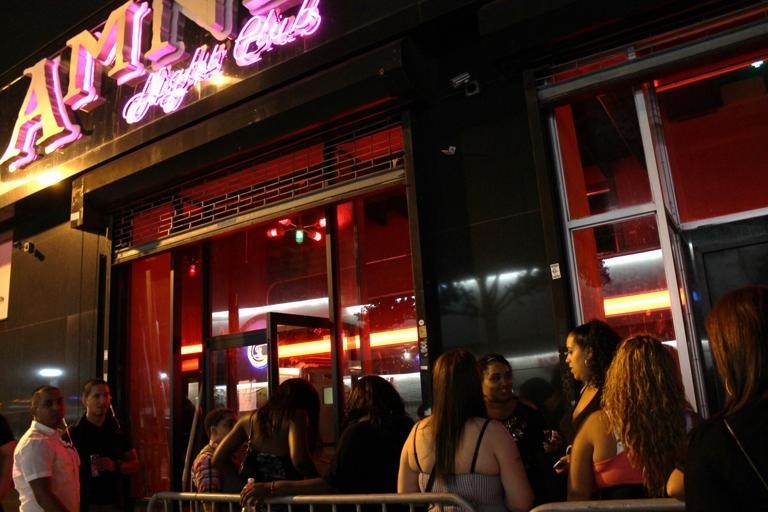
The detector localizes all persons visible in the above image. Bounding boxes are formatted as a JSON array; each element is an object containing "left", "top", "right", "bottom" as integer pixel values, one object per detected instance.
[
  {"left": 63, "top": 378, "right": 140, "bottom": 511},
  {"left": 12, "top": 385, "right": 81, "bottom": 511},
  {"left": 0, "top": 411, "right": 19, "bottom": 512}
]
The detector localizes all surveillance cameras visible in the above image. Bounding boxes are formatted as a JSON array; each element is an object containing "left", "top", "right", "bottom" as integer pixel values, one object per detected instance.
[
  {"left": 450, "top": 72, "right": 472, "bottom": 89},
  {"left": 13, "top": 241, "right": 23, "bottom": 248}
]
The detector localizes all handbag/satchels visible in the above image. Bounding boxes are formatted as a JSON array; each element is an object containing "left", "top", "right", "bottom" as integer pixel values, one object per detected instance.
[{"left": 239, "top": 440, "right": 295, "bottom": 482}]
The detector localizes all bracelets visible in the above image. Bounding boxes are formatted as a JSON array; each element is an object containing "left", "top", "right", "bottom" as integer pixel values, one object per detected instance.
[{"left": 114, "top": 462, "right": 121, "bottom": 475}]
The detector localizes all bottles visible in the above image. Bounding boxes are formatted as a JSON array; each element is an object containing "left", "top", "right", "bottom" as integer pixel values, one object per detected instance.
[{"left": 240, "top": 477, "right": 256, "bottom": 512}]
